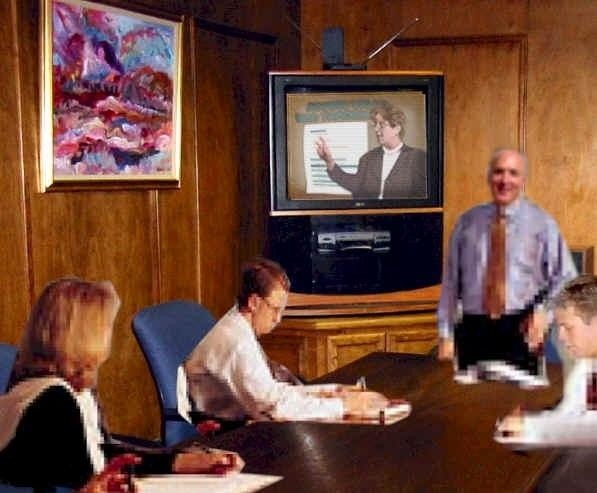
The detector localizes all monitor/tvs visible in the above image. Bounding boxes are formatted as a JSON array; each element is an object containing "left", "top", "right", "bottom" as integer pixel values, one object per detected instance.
[{"left": 269, "top": 70, "right": 444, "bottom": 216}]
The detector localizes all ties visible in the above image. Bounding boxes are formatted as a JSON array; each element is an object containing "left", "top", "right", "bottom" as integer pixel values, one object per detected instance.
[{"left": 481, "top": 205, "right": 507, "bottom": 320}]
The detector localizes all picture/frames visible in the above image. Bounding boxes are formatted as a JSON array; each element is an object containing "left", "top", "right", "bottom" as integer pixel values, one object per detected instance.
[{"left": 36, "top": 0, "right": 185, "bottom": 192}]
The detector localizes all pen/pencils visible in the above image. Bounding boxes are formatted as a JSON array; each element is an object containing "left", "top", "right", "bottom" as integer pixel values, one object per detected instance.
[
  {"left": 193, "top": 441, "right": 211, "bottom": 454},
  {"left": 128, "top": 464, "right": 135, "bottom": 493}
]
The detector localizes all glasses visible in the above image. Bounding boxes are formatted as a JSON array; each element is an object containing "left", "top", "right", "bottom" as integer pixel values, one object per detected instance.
[{"left": 371, "top": 120, "right": 392, "bottom": 129}]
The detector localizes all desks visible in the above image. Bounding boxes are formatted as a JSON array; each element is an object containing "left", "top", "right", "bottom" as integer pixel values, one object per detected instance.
[{"left": 115, "top": 349, "right": 596, "bottom": 491}]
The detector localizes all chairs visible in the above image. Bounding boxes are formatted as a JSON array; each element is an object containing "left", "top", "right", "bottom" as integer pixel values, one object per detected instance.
[
  {"left": 0, "top": 339, "right": 157, "bottom": 493},
  {"left": 132, "top": 297, "right": 272, "bottom": 447}
]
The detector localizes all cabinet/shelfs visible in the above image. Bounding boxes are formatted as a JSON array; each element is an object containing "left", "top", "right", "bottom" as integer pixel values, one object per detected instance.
[{"left": 253, "top": 282, "right": 480, "bottom": 394}]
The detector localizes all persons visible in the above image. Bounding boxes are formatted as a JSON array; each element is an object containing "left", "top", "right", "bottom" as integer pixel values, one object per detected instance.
[
  {"left": 437, "top": 149, "right": 579, "bottom": 373},
  {"left": 495, "top": 273, "right": 597, "bottom": 445},
  {"left": 314, "top": 105, "right": 426, "bottom": 198},
  {"left": 185, "top": 259, "right": 390, "bottom": 422},
  {"left": 0, "top": 454, "right": 141, "bottom": 493},
  {"left": 0, "top": 277, "right": 245, "bottom": 485}
]
